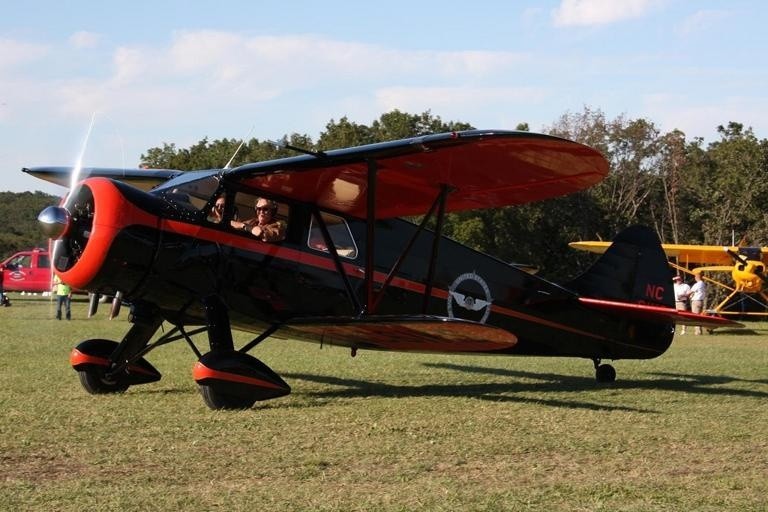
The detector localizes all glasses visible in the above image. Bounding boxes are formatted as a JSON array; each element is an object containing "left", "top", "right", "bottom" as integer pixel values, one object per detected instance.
[{"left": 255, "top": 206, "right": 268, "bottom": 211}]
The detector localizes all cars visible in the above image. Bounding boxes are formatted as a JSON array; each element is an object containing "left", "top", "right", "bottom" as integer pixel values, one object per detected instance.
[{"left": 0, "top": 247, "right": 53, "bottom": 292}]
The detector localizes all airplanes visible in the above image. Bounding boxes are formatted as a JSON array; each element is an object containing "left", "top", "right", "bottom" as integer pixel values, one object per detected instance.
[
  {"left": 568, "top": 233, "right": 768, "bottom": 318},
  {"left": 21, "top": 130, "right": 747, "bottom": 410}
]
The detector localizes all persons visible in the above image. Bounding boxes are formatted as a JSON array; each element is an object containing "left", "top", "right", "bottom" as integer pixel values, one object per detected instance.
[
  {"left": 53, "top": 274, "right": 72, "bottom": 319},
  {"left": 207, "top": 196, "right": 284, "bottom": 242},
  {"left": 673, "top": 272, "right": 707, "bottom": 335}
]
